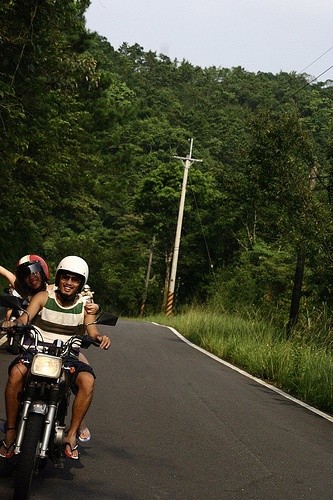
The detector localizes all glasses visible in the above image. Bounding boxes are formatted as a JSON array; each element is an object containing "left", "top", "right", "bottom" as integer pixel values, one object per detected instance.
[{"left": 59, "top": 273, "right": 82, "bottom": 282}]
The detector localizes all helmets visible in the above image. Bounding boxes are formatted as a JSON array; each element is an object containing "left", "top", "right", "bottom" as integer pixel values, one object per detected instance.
[
  {"left": 54, "top": 256, "right": 89, "bottom": 294},
  {"left": 84, "top": 284, "right": 89, "bottom": 292},
  {"left": 16, "top": 255, "right": 50, "bottom": 292}
]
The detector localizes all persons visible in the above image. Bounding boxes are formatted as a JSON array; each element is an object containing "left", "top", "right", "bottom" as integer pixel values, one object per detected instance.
[{"left": 0, "top": 254, "right": 111, "bottom": 459}]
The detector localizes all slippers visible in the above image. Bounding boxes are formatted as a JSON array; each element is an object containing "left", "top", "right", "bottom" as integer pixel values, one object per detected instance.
[
  {"left": 76, "top": 422, "right": 91, "bottom": 442},
  {"left": 0, "top": 439, "right": 17, "bottom": 458},
  {"left": 61, "top": 436, "right": 79, "bottom": 460}
]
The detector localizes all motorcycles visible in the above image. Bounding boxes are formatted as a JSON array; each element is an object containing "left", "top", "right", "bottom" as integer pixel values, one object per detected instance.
[{"left": 0, "top": 294, "right": 118, "bottom": 500}]
